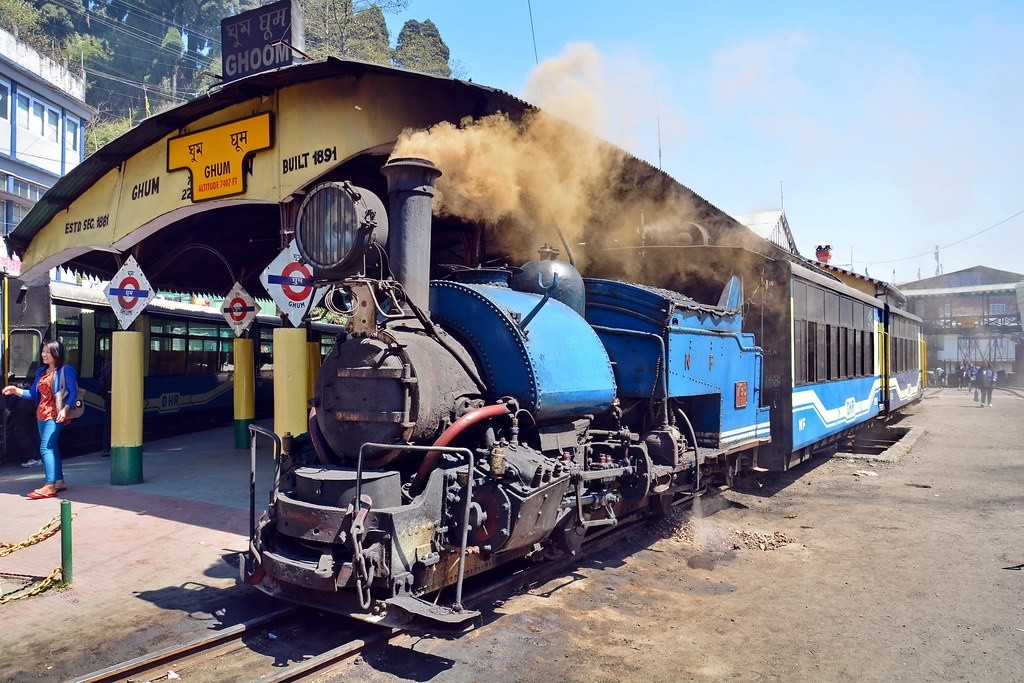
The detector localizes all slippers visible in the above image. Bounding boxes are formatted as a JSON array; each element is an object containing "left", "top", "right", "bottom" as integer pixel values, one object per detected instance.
[
  {"left": 28, "top": 489, "right": 57, "bottom": 498},
  {"left": 56, "top": 488, "right": 67, "bottom": 493}
]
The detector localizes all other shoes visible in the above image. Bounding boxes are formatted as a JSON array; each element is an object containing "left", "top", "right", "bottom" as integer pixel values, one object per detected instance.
[
  {"left": 981, "top": 404, "right": 986, "bottom": 407},
  {"left": 988, "top": 403, "right": 993, "bottom": 407}
]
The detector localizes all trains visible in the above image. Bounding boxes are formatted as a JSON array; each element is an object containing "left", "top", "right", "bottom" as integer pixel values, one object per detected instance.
[
  {"left": 222, "top": 155, "right": 928, "bottom": 634},
  {"left": 0, "top": 275, "right": 346, "bottom": 463}
]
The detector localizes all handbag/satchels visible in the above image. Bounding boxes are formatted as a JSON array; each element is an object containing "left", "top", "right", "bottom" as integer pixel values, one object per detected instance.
[
  {"left": 974, "top": 392, "right": 980, "bottom": 402},
  {"left": 56, "top": 364, "right": 86, "bottom": 419}
]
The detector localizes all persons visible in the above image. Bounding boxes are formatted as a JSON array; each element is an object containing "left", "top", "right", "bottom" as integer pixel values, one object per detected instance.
[
  {"left": 954, "top": 360, "right": 997, "bottom": 407},
  {"left": 0, "top": 338, "right": 79, "bottom": 500},
  {"left": 926, "top": 365, "right": 944, "bottom": 387}
]
